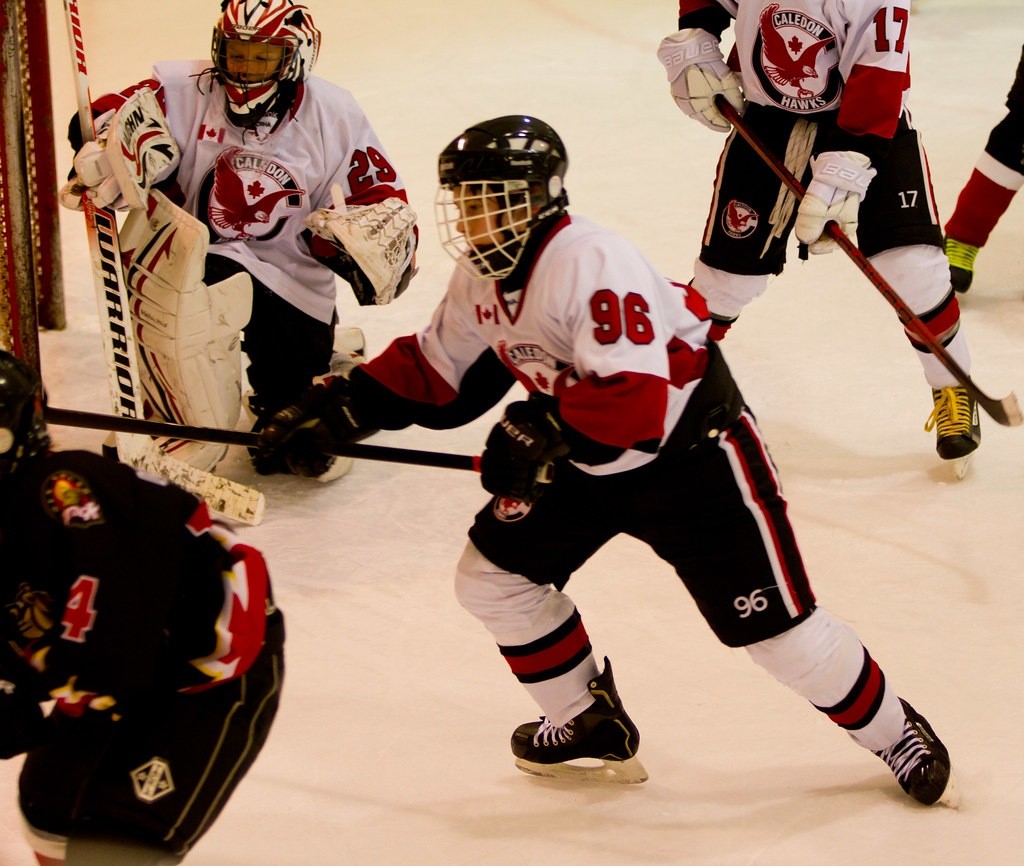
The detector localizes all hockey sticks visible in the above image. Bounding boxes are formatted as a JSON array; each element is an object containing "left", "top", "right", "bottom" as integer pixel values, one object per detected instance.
[
  {"left": 60, "top": 1, "right": 266, "bottom": 525},
  {"left": 42, "top": 405, "right": 554, "bottom": 482},
  {"left": 715, "top": 94, "right": 1024, "bottom": 428}
]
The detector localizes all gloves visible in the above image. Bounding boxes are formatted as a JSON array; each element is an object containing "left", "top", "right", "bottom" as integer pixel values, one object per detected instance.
[
  {"left": 248, "top": 374, "right": 379, "bottom": 474},
  {"left": 794, "top": 151, "right": 876, "bottom": 254},
  {"left": 658, "top": 28, "right": 745, "bottom": 132},
  {"left": 478, "top": 392, "right": 575, "bottom": 502}
]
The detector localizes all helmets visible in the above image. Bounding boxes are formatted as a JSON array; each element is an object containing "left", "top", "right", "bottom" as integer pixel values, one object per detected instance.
[
  {"left": 215, "top": 0, "right": 322, "bottom": 130},
  {"left": 438, "top": 115, "right": 568, "bottom": 207}
]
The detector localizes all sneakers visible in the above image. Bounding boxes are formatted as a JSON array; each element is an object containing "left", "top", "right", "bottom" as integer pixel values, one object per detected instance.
[
  {"left": 510, "top": 656, "right": 649, "bottom": 784},
  {"left": 925, "top": 375, "right": 981, "bottom": 480},
  {"left": 945, "top": 234, "right": 978, "bottom": 305},
  {"left": 871, "top": 697, "right": 962, "bottom": 810}
]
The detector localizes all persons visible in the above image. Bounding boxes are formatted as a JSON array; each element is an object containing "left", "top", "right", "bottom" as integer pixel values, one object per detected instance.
[
  {"left": 945, "top": 43, "right": 1024, "bottom": 293},
  {"left": 248, "top": 115, "right": 951, "bottom": 807},
  {"left": 66, "top": 0, "right": 419, "bottom": 483},
  {"left": 0, "top": 350, "right": 285, "bottom": 866},
  {"left": 657, "top": 0, "right": 982, "bottom": 481}
]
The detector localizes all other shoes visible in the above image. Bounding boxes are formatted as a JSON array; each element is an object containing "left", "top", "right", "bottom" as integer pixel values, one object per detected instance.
[{"left": 333, "top": 325, "right": 367, "bottom": 371}]
